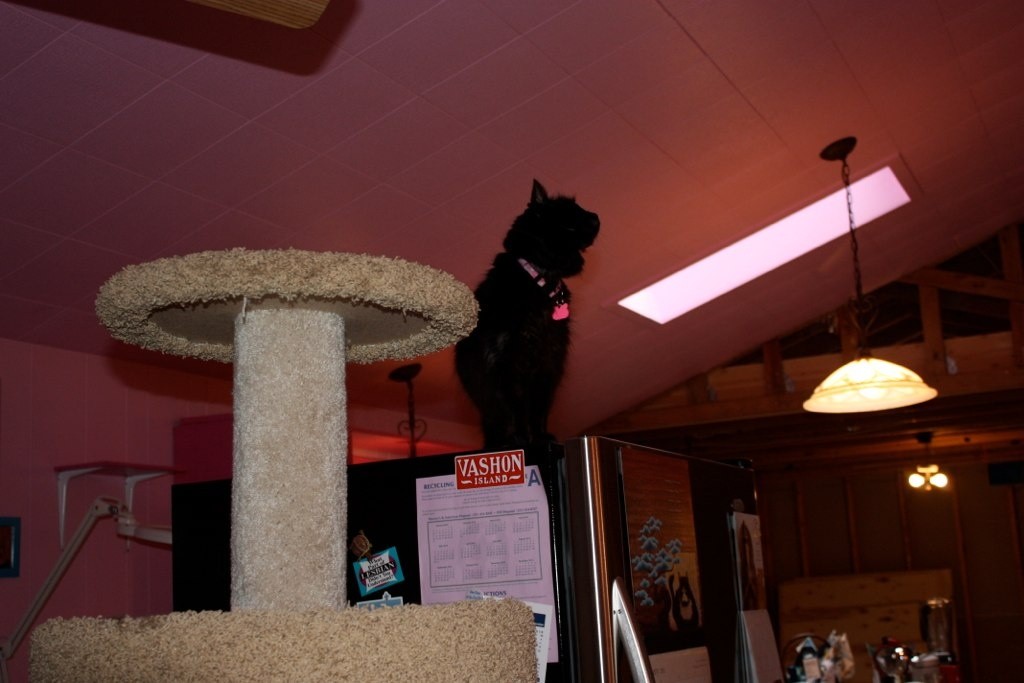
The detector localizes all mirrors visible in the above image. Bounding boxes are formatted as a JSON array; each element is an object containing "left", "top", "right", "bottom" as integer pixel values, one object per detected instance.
[{"left": 0, "top": 517, "right": 20, "bottom": 577}]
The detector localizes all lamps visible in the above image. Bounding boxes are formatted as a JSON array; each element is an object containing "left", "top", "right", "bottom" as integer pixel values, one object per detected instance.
[
  {"left": 908, "top": 430, "right": 948, "bottom": 491},
  {"left": 801, "top": 134, "right": 938, "bottom": 413}
]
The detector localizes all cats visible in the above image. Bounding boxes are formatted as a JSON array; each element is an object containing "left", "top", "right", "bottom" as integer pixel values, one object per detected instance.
[{"left": 455, "top": 178, "right": 602, "bottom": 464}]
